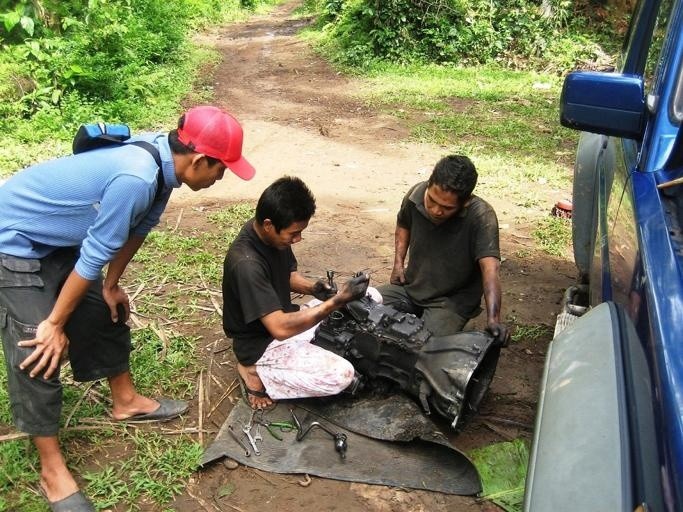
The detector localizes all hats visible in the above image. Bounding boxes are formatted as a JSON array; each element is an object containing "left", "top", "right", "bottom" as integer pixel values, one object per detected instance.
[{"left": 176, "top": 104, "right": 256, "bottom": 182}]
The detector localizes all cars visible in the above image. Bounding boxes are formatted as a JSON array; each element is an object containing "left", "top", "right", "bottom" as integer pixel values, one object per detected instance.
[{"left": 520, "top": 1, "right": 682, "bottom": 511}]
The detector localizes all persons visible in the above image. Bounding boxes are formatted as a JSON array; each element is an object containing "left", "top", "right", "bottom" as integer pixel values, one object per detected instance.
[
  {"left": 221, "top": 175, "right": 382, "bottom": 411},
  {"left": 372, "top": 154, "right": 512, "bottom": 350},
  {"left": 0, "top": 104, "right": 256, "bottom": 510}
]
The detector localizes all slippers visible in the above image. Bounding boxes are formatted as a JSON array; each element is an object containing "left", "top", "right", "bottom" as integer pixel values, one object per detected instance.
[
  {"left": 128, "top": 397, "right": 191, "bottom": 422},
  {"left": 37, "top": 484, "right": 96, "bottom": 512},
  {"left": 238, "top": 372, "right": 274, "bottom": 412}
]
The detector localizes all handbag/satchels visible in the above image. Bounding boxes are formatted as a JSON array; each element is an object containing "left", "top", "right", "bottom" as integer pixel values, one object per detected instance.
[{"left": 71, "top": 121, "right": 132, "bottom": 155}]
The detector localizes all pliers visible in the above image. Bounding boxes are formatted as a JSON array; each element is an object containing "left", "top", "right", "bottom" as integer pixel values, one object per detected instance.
[{"left": 255, "top": 417, "right": 297, "bottom": 441}]
